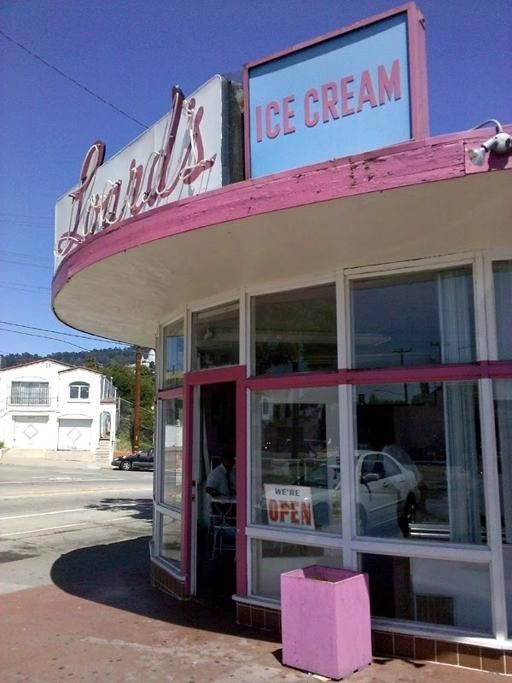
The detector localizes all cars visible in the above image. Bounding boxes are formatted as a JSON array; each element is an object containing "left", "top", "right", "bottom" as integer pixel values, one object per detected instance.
[
  {"left": 290, "top": 450, "right": 420, "bottom": 538},
  {"left": 112, "top": 448, "right": 155, "bottom": 471}
]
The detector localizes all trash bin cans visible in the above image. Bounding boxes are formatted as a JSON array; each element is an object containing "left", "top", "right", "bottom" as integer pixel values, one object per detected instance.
[{"left": 280, "top": 564, "right": 373, "bottom": 681}]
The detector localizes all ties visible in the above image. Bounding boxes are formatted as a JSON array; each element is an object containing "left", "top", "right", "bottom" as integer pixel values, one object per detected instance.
[{"left": 226, "top": 472, "right": 234, "bottom": 490}]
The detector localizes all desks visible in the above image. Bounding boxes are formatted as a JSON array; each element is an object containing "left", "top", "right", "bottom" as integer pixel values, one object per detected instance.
[{"left": 212, "top": 493, "right": 237, "bottom": 554}]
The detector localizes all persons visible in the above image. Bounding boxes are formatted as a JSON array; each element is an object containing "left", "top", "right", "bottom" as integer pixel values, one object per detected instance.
[{"left": 205, "top": 451, "right": 236, "bottom": 526}]
